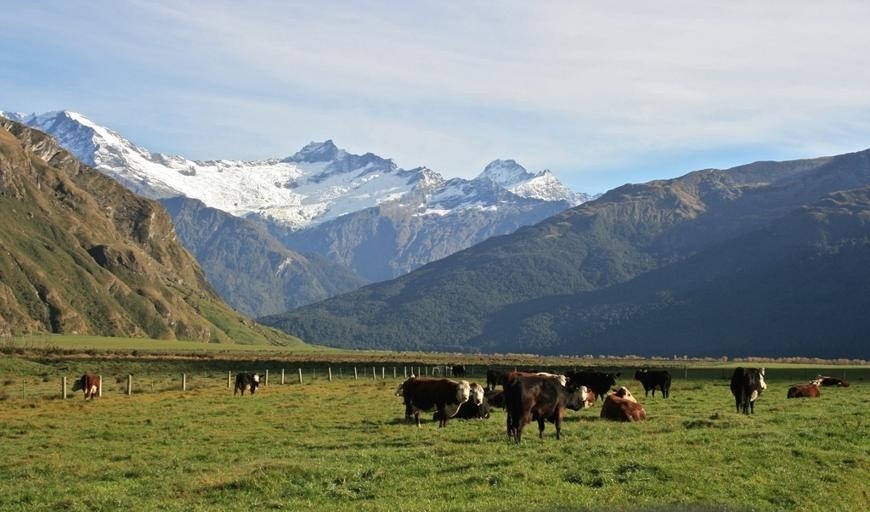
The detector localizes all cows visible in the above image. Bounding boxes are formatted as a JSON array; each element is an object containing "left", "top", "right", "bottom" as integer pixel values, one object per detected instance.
[
  {"left": 401, "top": 368, "right": 623, "bottom": 443},
  {"left": 634, "top": 367, "right": 672, "bottom": 399},
  {"left": 729, "top": 367, "right": 767, "bottom": 414},
  {"left": 787, "top": 378, "right": 821, "bottom": 397},
  {"left": 71, "top": 373, "right": 102, "bottom": 401},
  {"left": 232, "top": 371, "right": 264, "bottom": 396},
  {"left": 602, "top": 387, "right": 648, "bottom": 422},
  {"left": 817, "top": 374, "right": 850, "bottom": 388}
]
